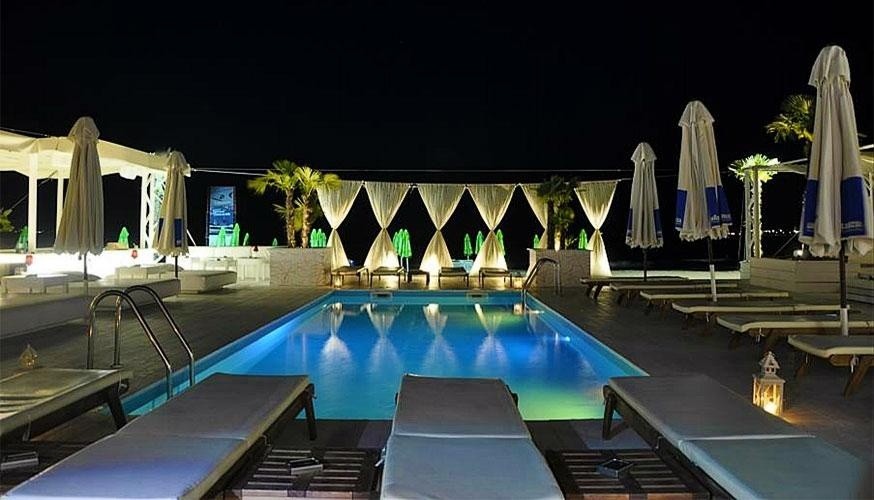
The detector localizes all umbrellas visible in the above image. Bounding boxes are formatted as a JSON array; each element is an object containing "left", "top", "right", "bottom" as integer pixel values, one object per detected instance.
[
  {"left": 12, "top": 225, "right": 30, "bottom": 250},
  {"left": 576, "top": 226, "right": 587, "bottom": 250},
  {"left": 671, "top": 99, "right": 734, "bottom": 305},
  {"left": 269, "top": 237, "right": 278, "bottom": 246},
  {"left": 623, "top": 142, "right": 666, "bottom": 282},
  {"left": 242, "top": 231, "right": 250, "bottom": 246},
  {"left": 307, "top": 224, "right": 511, "bottom": 283},
  {"left": 148, "top": 150, "right": 194, "bottom": 281},
  {"left": 530, "top": 234, "right": 540, "bottom": 247},
  {"left": 48, "top": 116, "right": 109, "bottom": 284},
  {"left": 115, "top": 226, "right": 130, "bottom": 249},
  {"left": 227, "top": 222, "right": 239, "bottom": 246},
  {"left": 798, "top": 44, "right": 873, "bottom": 337},
  {"left": 214, "top": 225, "right": 225, "bottom": 247}
]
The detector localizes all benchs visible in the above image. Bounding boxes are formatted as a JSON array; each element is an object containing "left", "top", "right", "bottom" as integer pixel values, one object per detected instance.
[
  {"left": 578, "top": 275, "right": 873, "bottom": 398},
  {"left": 0, "top": 260, "right": 236, "bottom": 337},
  {"left": 330, "top": 266, "right": 511, "bottom": 289}
]
